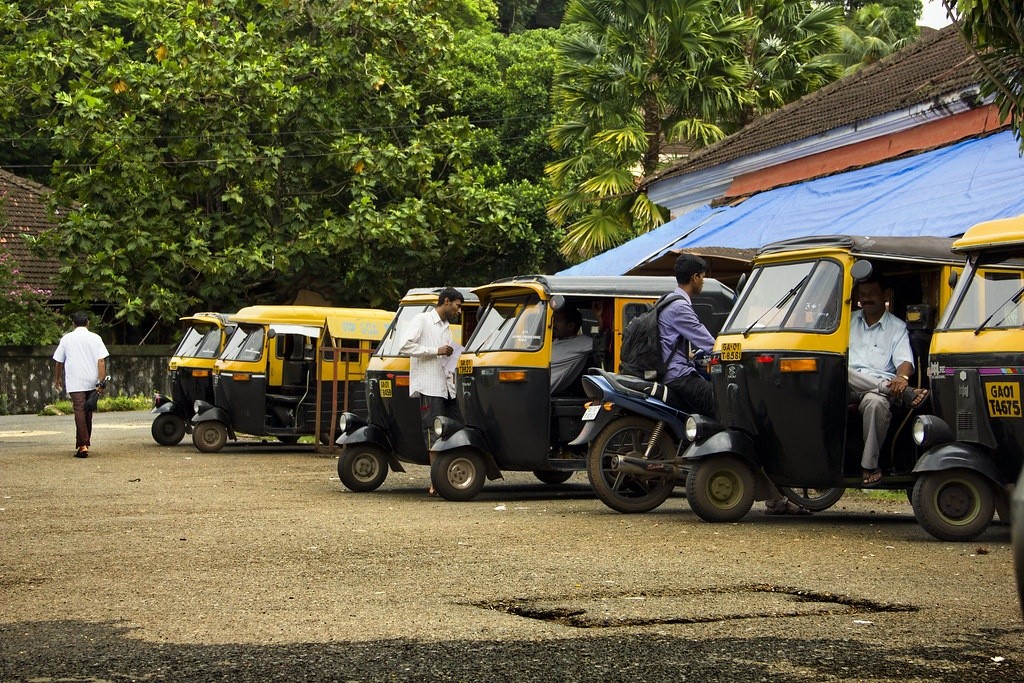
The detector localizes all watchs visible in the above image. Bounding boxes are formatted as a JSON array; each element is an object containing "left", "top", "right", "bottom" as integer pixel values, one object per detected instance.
[{"left": 898, "top": 375, "right": 909, "bottom": 382}]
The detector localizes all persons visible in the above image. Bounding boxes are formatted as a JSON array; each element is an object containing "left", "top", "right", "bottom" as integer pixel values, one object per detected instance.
[
  {"left": 550, "top": 297, "right": 604, "bottom": 395},
  {"left": 399, "top": 286, "right": 464, "bottom": 497},
  {"left": 847, "top": 275, "right": 931, "bottom": 488},
  {"left": 657, "top": 254, "right": 721, "bottom": 421},
  {"left": 53, "top": 312, "right": 109, "bottom": 458}
]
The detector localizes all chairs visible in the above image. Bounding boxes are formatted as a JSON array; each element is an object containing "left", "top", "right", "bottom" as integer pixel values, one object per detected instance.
[
  {"left": 266, "top": 360, "right": 308, "bottom": 404},
  {"left": 550, "top": 330, "right": 611, "bottom": 407}
]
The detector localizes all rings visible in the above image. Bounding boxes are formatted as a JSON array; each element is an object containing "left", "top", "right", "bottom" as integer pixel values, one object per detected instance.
[{"left": 900, "top": 390, "right": 903, "bottom": 393}]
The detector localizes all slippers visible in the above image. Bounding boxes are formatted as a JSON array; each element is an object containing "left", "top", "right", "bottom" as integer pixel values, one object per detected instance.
[
  {"left": 861, "top": 467, "right": 884, "bottom": 488},
  {"left": 892, "top": 386, "right": 930, "bottom": 409},
  {"left": 74, "top": 447, "right": 89, "bottom": 458}
]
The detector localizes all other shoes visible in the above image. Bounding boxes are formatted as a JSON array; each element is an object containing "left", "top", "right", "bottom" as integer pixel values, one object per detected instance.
[
  {"left": 774, "top": 496, "right": 813, "bottom": 515},
  {"left": 428, "top": 490, "right": 440, "bottom": 498}
]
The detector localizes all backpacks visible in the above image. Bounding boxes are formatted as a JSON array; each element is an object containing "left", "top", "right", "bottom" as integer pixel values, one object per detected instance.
[{"left": 618, "top": 292, "right": 690, "bottom": 381}]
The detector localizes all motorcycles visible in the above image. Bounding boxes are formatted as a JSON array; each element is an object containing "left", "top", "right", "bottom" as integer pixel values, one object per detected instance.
[
  {"left": 335, "top": 285, "right": 542, "bottom": 494},
  {"left": 567, "top": 344, "right": 714, "bottom": 515},
  {"left": 431, "top": 274, "right": 736, "bottom": 503},
  {"left": 679, "top": 236, "right": 1024, "bottom": 524},
  {"left": 150, "top": 304, "right": 395, "bottom": 452},
  {"left": 910, "top": 215, "right": 1024, "bottom": 543}
]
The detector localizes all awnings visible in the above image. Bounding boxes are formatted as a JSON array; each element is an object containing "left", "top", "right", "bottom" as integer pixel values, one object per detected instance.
[{"left": 553, "top": 94, "right": 1024, "bottom": 276}]
